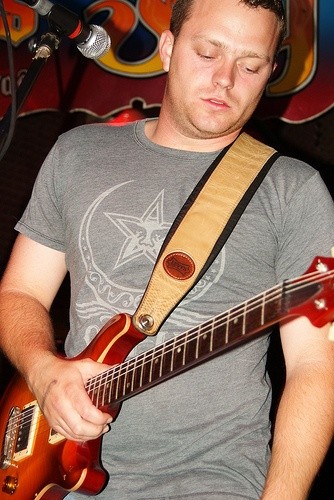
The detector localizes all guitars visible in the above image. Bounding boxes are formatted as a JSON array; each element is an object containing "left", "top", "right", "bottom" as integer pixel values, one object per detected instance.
[{"left": 2, "top": 246, "right": 334, "bottom": 500}]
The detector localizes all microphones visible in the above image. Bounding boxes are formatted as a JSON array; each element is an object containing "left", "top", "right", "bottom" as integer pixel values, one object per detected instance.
[{"left": 19, "top": 0, "right": 112, "bottom": 60}]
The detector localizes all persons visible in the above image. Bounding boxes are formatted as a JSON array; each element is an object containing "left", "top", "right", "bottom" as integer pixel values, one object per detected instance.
[{"left": 0, "top": 0, "right": 334, "bottom": 500}]
